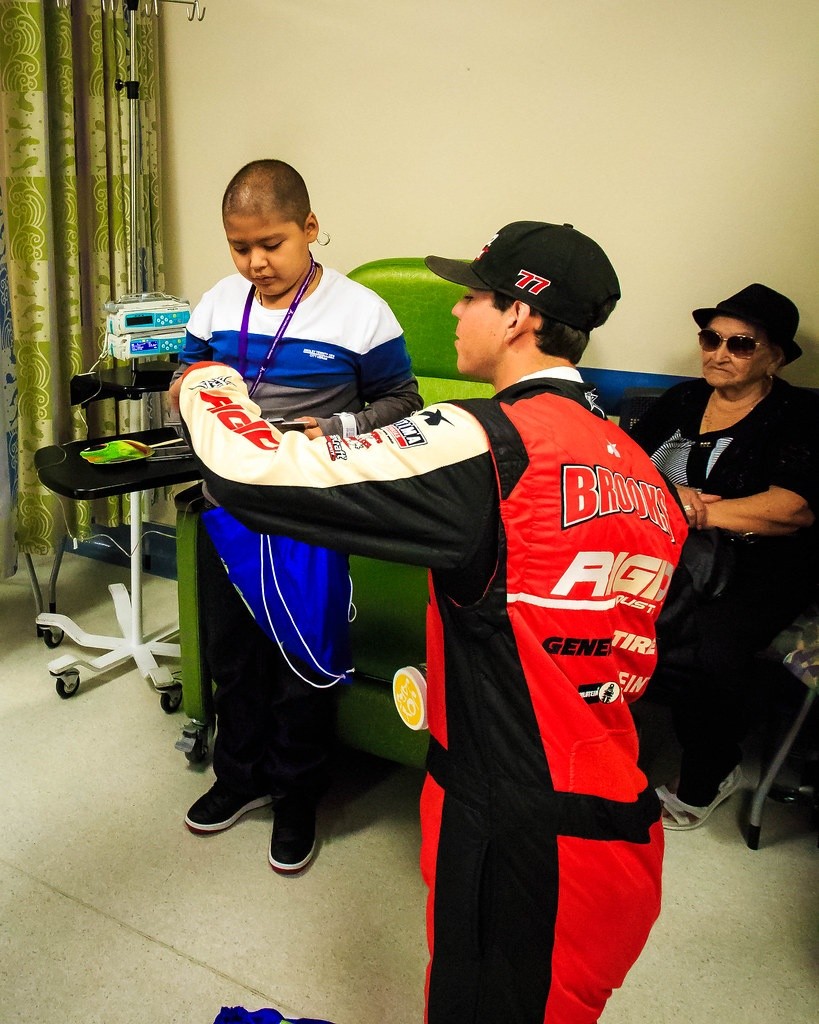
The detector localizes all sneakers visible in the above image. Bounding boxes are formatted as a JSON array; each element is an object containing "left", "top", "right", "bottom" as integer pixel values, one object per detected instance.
[
  {"left": 184, "top": 781, "right": 272, "bottom": 833},
  {"left": 268, "top": 807, "right": 317, "bottom": 870}
]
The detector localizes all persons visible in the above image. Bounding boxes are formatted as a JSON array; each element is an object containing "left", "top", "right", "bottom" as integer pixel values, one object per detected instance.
[
  {"left": 611, "top": 281, "right": 818, "bottom": 835},
  {"left": 167, "top": 158, "right": 426, "bottom": 876},
  {"left": 166, "top": 219, "right": 698, "bottom": 1024}
]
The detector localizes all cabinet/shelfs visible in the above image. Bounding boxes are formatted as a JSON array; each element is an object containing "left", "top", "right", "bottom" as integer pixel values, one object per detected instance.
[{"left": 34, "top": 355, "right": 202, "bottom": 714}]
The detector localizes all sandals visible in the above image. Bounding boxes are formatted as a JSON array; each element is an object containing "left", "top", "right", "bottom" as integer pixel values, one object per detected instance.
[{"left": 656, "top": 765, "right": 742, "bottom": 830}]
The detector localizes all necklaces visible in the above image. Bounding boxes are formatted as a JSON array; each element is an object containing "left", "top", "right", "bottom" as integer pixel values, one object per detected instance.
[
  {"left": 705, "top": 374, "right": 776, "bottom": 433},
  {"left": 257, "top": 263, "right": 321, "bottom": 308}
]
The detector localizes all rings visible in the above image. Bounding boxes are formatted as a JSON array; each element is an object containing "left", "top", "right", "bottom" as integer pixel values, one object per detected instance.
[{"left": 682, "top": 504, "right": 694, "bottom": 512}]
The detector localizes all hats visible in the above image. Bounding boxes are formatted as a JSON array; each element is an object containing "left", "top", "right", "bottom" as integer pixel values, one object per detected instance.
[
  {"left": 692, "top": 282, "right": 802, "bottom": 368},
  {"left": 424, "top": 221, "right": 622, "bottom": 332}
]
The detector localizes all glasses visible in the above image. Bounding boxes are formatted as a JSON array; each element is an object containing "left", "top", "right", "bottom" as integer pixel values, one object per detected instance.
[{"left": 697, "top": 329, "right": 767, "bottom": 360}]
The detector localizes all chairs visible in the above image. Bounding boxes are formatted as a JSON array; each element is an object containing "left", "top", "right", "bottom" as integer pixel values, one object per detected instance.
[
  {"left": 747, "top": 611, "right": 819, "bottom": 850},
  {"left": 175, "top": 257, "right": 496, "bottom": 782}
]
